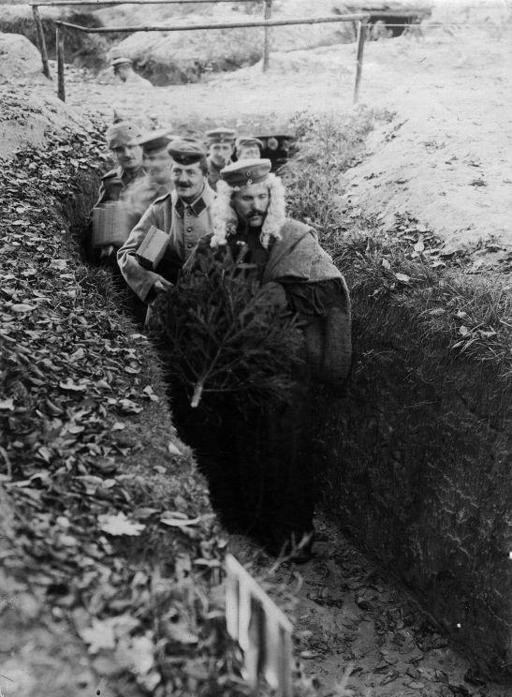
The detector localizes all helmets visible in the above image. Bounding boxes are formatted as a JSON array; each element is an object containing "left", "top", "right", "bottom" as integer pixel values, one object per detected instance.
[{"left": 108, "top": 122, "right": 140, "bottom": 149}]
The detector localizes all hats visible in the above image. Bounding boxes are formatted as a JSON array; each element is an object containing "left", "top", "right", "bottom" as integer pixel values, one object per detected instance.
[
  {"left": 220, "top": 159, "right": 273, "bottom": 186},
  {"left": 138, "top": 132, "right": 170, "bottom": 153},
  {"left": 112, "top": 58, "right": 133, "bottom": 68},
  {"left": 168, "top": 130, "right": 263, "bottom": 164}
]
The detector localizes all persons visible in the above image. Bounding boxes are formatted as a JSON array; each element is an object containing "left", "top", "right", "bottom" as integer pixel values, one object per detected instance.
[
  {"left": 95, "top": 122, "right": 264, "bottom": 333},
  {"left": 143, "top": 159, "right": 354, "bottom": 565}
]
